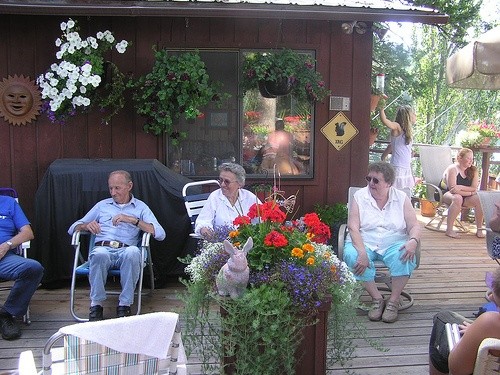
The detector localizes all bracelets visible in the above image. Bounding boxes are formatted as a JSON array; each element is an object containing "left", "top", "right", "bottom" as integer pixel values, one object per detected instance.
[
  {"left": 471, "top": 191, "right": 473, "bottom": 196},
  {"left": 410, "top": 237, "right": 419, "bottom": 244},
  {"left": 136, "top": 218, "right": 140, "bottom": 226}
]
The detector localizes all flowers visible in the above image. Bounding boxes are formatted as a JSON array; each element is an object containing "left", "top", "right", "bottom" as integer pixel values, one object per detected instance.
[
  {"left": 34, "top": 17, "right": 130, "bottom": 125},
  {"left": 413, "top": 177, "right": 427, "bottom": 197},
  {"left": 243, "top": 49, "right": 325, "bottom": 104},
  {"left": 454, "top": 117, "right": 500, "bottom": 148},
  {"left": 370, "top": 117, "right": 381, "bottom": 130},
  {"left": 132, "top": 41, "right": 232, "bottom": 139},
  {"left": 171, "top": 162, "right": 366, "bottom": 375},
  {"left": 371, "top": 85, "right": 389, "bottom": 99}
]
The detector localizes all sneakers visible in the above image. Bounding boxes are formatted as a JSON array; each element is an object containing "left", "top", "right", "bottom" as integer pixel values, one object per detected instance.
[
  {"left": 0, "top": 312, "right": 22, "bottom": 340},
  {"left": 116, "top": 305, "right": 131, "bottom": 318},
  {"left": 88, "top": 305, "right": 103, "bottom": 322},
  {"left": 367, "top": 295, "right": 400, "bottom": 322}
]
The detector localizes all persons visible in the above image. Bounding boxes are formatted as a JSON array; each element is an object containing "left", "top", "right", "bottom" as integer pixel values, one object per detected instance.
[
  {"left": 380, "top": 94, "right": 417, "bottom": 204},
  {"left": 68, "top": 170, "right": 168, "bottom": 322},
  {"left": 434, "top": 147, "right": 486, "bottom": 240},
  {"left": 276, "top": 120, "right": 285, "bottom": 131},
  {"left": 343, "top": 161, "right": 422, "bottom": 323},
  {"left": 195, "top": 162, "right": 266, "bottom": 242},
  {"left": 428, "top": 265, "right": 500, "bottom": 375},
  {"left": 1, "top": 195, "right": 44, "bottom": 342}
]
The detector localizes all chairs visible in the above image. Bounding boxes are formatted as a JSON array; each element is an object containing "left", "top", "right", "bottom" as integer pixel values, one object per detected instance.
[
  {"left": 68, "top": 230, "right": 155, "bottom": 322},
  {"left": 182, "top": 179, "right": 224, "bottom": 241},
  {"left": 17, "top": 312, "right": 189, "bottom": 375},
  {"left": 0, "top": 188, "right": 34, "bottom": 325},
  {"left": 419, "top": 145, "right": 472, "bottom": 234},
  {"left": 443, "top": 322, "right": 500, "bottom": 375},
  {"left": 337, "top": 186, "right": 421, "bottom": 311},
  {"left": 476, "top": 190, "right": 500, "bottom": 277}
]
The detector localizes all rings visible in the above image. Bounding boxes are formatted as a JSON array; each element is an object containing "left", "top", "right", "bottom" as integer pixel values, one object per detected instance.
[{"left": 455, "top": 191, "right": 457, "bottom": 192}]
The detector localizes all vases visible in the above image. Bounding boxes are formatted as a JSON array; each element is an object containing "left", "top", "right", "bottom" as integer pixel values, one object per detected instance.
[
  {"left": 219, "top": 293, "right": 331, "bottom": 375},
  {"left": 480, "top": 137, "right": 491, "bottom": 146},
  {"left": 370, "top": 128, "right": 379, "bottom": 145},
  {"left": 259, "top": 75, "right": 294, "bottom": 98},
  {"left": 371, "top": 93, "right": 380, "bottom": 112},
  {"left": 420, "top": 198, "right": 437, "bottom": 216}
]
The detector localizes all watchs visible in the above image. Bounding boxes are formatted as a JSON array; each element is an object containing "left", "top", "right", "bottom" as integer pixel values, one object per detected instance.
[{"left": 6, "top": 240, "right": 14, "bottom": 251}]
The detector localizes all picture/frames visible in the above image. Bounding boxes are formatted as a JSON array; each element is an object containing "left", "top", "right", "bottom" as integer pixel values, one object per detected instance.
[{"left": 240, "top": 45, "right": 316, "bottom": 181}]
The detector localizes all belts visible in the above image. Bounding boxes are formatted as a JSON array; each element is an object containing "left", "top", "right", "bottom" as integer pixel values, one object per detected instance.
[{"left": 95, "top": 241, "right": 129, "bottom": 248}]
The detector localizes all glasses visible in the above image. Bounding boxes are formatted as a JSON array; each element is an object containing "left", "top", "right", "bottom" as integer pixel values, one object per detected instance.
[
  {"left": 216, "top": 178, "right": 237, "bottom": 186},
  {"left": 364, "top": 175, "right": 384, "bottom": 184}
]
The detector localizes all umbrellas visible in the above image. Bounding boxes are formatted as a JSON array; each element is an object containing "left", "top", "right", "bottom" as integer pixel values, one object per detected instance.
[{"left": 446, "top": 23, "right": 500, "bottom": 91}]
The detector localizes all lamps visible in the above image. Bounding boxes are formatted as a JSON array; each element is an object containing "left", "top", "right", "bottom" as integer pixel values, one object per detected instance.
[
  {"left": 371, "top": 25, "right": 388, "bottom": 39},
  {"left": 341, "top": 21, "right": 358, "bottom": 35},
  {"left": 355, "top": 22, "right": 368, "bottom": 35}
]
